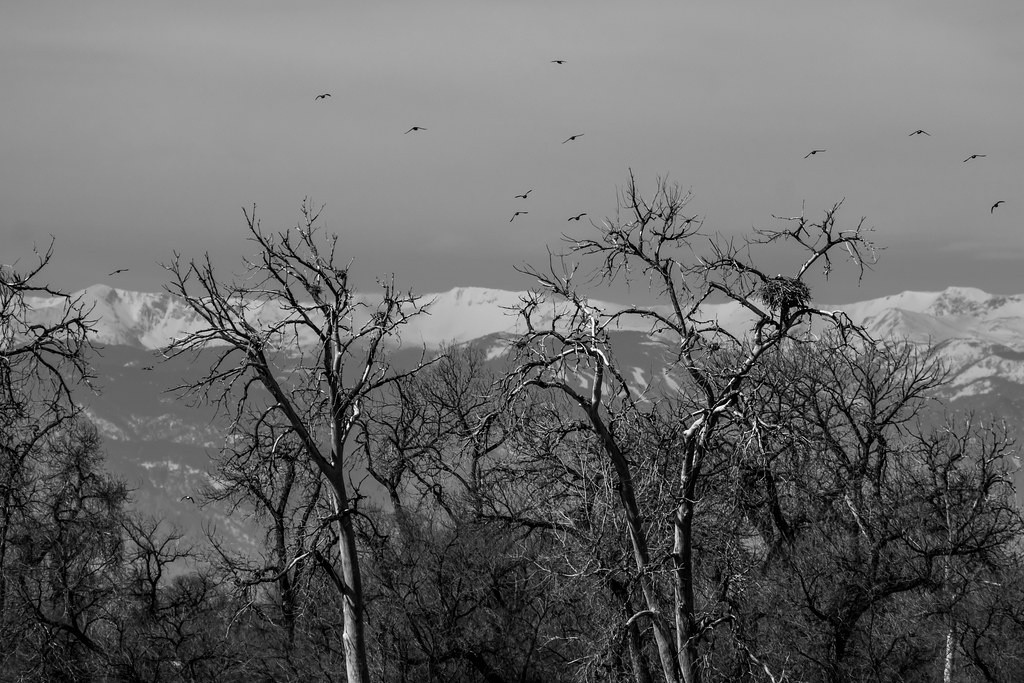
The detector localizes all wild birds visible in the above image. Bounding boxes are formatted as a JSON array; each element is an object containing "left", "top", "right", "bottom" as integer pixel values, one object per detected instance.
[
  {"left": 314, "top": 93, "right": 331, "bottom": 101},
  {"left": 963, "top": 154, "right": 986, "bottom": 163},
  {"left": 108, "top": 268, "right": 129, "bottom": 277},
  {"left": 804, "top": 150, "right": 826, "bottom": 159},
  {"left": 510, "top": 211, "right": 528, "bottom": 222},
  {"left": 562, "top": 134, "right": 584, "bottom": 144},
  {"left": 181, "top": 495, "right": 195, "bottom": 503},
  {"left": 990, "top": 200, "right": 1005, "bottom": 214},
  {"left": 909, "top": 129, "right": 932, "bottom": 137},
  {"left": 514, "top": 190, "right": 532, "bottom": 198},
  {"left": 404, "top": 126, "right": 426, "bottom": 135},
  {"left": 568, "top": 212, "right": 587, "bottom": 222},
  {"left": 550, "top": 60, "right": 567, "bottom": 65}
]
